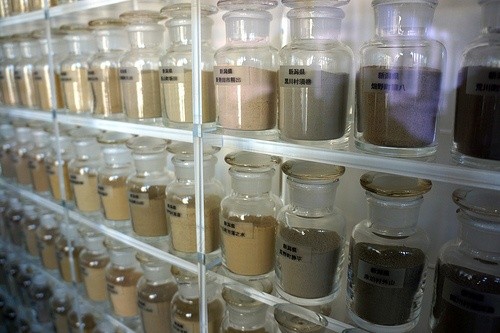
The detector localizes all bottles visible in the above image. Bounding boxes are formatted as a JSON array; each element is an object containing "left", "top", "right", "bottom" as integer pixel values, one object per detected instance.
[
  {"left": 223, "top": 152, "right": 283, "bottom": 278},
  {"left": 275, "top": 160, "right": 347, "bottom": 306},
  {"left": 127, "top": 137, "right": 168, "bottom": 238},
  {"left": 355, "top": 0, "right": 446, "bottom": 154},
  {"left": 166, "top": 145, "right": 220, "bottom": 254},
  {"left": 214, "top": 0, "right": 279, "bottom": 131},
  {"left": 120, "top": 11, "right": 159, "bottom": 120},
  {"left": 349, "top": 171, "right": 432, "bottom": 333},
  {"left": 449, "top": 0, "right": 500, "bottom": 170},
  {"left": 0, "top": 186, "right": 330, "bottom": 333},
  {"left": 430, "top": 187, "right": 500, "bottom": 333},
  {"left": 279, "top": 0, "right": 353, "bottom": 146},
  {"left": 0, "top": 0, "right": 131, "bottom": 222},
  {"left": 160, "top": 4, "right": 214, "bottom": 124}
]
